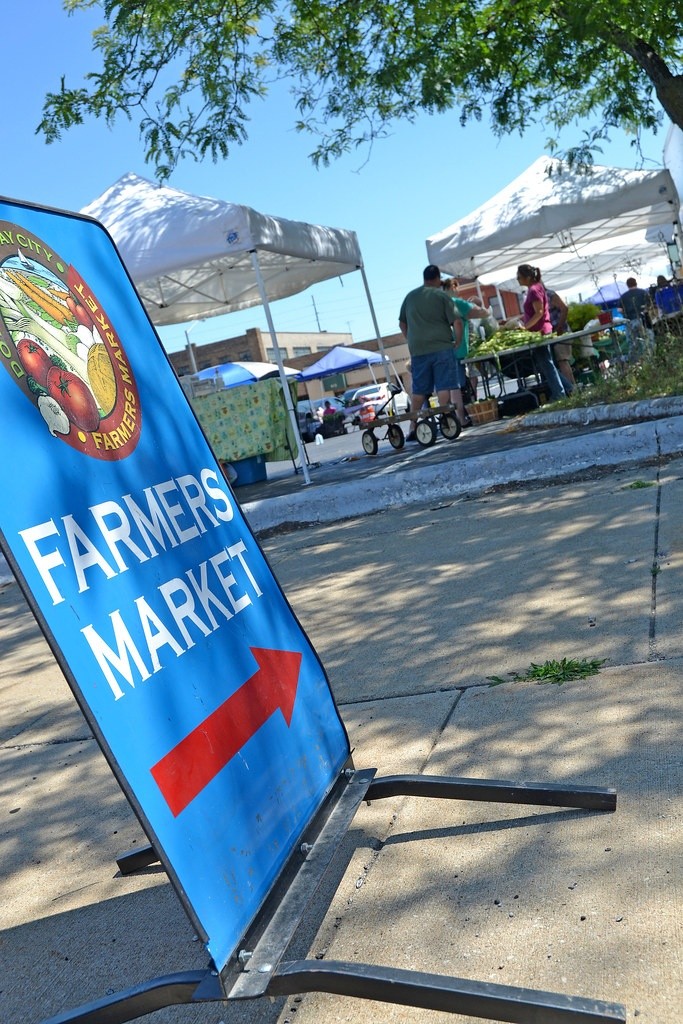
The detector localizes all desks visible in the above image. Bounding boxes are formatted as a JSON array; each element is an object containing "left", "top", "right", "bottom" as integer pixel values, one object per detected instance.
[
  {"left": 460, "top": 319, "right": 630, "bottom": 413},
  {"left": 188, "top": 376, "right": 300, "bottom": 464},
  {"left": 343, "top": 398, "right": 387, "bottom": 434}
]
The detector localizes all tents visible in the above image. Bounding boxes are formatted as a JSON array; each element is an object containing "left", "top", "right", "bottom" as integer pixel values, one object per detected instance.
[
  {"left": 298, "top": 346, "right": 409, "bottom": 416},
  {"left": 584, "top": 281, "right": 629, "bottom": 309},
  {"left": 426, "top": 156, "right": 683, "bottom": 315},
  {"left": 80, "top": 171, "right": 397, "bottom": 487}
]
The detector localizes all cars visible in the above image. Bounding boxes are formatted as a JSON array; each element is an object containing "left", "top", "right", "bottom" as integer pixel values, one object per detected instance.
[
  {"left": 296, "top": 389, "right": 357, "bottom": 443},
  {"left": 352, "top": 382, "right": 411, "bottom": 413}
]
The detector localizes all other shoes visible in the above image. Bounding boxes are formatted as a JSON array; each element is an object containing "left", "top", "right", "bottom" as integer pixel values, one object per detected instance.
[
  {"left": 461, "top": 420, "right": 472, "bottom": 428},
  {"left": 406, "top": 431, "right": 417, "bottom": 441}
]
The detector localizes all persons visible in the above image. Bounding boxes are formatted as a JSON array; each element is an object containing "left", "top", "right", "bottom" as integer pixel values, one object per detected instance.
[
  {"left": 323, "top": 401, "right": 336, "bottom": 416},
  {"left": 610, "top": 307, "right": 623, "bottom": 318},
  {"left": 439, "top": 278, "right": 490, "bottom": 428},
  {"left": 465, "top": 295, "right": 483, "bottom": 395},
  {"left": 399, "top": 265, "right": 462, "bottom": 441},
  {"left": 517, "top": 265, "right": 573, "bottom": 403},
  {"left": 618, "top": 278, "right": 653, "bottom": 363},
  {"left": 655, "top": 276, "right": 680, "bottom": 315},
  {"left": 541, "top": 279, "right": 580, "bottom": 396}
]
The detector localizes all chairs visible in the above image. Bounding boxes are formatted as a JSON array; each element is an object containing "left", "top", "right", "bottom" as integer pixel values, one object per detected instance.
[{"left": 650, "top": 286, "right": 683, "bottom": 336}]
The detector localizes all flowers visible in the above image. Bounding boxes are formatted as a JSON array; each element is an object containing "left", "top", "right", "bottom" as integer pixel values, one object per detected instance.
[{"left": 334, "top": 394, "right": 386, "bottom": 408}]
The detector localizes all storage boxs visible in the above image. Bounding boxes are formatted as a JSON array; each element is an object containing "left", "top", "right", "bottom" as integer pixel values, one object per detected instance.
[
  {"left": 465, "top": 398, "right": 498, "bottom": 425},
  {"left": 223, "top": 453, "right": 268, "bottom": 487}
]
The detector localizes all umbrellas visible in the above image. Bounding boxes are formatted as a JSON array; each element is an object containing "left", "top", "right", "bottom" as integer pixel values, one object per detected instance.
[{"left": 190, "top": 361, "right": 300, "bottom": 388}]
